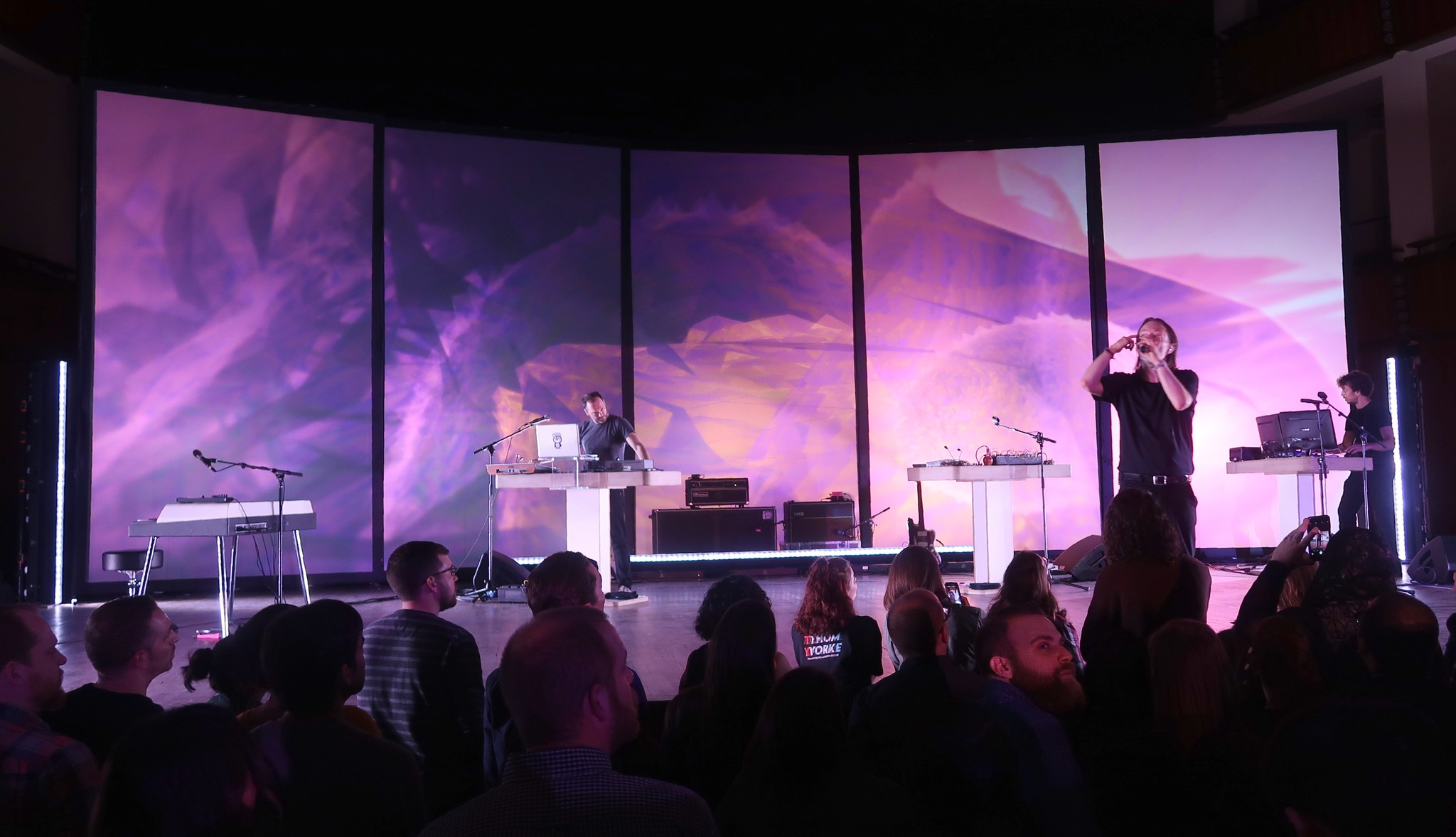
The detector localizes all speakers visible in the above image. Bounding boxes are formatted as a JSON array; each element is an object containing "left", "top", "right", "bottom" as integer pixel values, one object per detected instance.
[
  {"left": 1054, "top": 534, "right": 1107, "bottom": 582},
  {"left": 1407, "top": 535, "right": 1456, "bottom": 585},
  {"left": 473, "top": 550, "right": 532, "bottom": 601},
  {"left": 685, "top": 478, "right": 749, "bottom": 509},
  {"left": 783, "top": 500, "right": 855, "bottom": 542},
  {"left": 648, "top": 506, "right": 778, "bottom": 554}
]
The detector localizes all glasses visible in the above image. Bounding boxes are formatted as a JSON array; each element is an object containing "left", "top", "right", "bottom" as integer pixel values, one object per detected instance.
[
  {"left": 421, "top": 566, "right": 457, "bottom": 587},
  {"left": 938, "top": 608, "right": 951, "bottom": 635}
]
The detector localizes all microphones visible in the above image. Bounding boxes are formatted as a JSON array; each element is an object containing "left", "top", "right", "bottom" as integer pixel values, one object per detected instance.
[
  {"left": 1300, "top": 398, "right": 1322, "bottom": 405},
  {"left": 1141, "top": 346, "right": 1151, "bottom": 353},
  {"left": 944, "top": 446, "right": 954, "bottom": 460},
  {"left": 834, "top": 528, "right": 852, "bottom": 538},
  {"left": 957, "top": 448, "right": 962, "bottom": 461},
  {"left": 1318, "top": 391, "right": 1329, "bottom": 404},
  {"left": 192, "top": 450, "right": 215, "bottom": 472},
  {"left": 528, "top": 414, "right": 551, "bottom": 426}
]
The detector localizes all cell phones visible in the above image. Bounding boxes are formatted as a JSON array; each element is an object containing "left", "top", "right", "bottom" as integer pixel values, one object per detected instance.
[
  {"left": 1307, "top": 514, "right": 1332, "bottom": 560},
  {"left": 945, "top": 582, "right": 963, "bottom": 606}
]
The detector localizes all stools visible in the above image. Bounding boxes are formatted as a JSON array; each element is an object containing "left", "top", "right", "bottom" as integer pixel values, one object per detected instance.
[{"left": 101, "top": 549, "right": 178, "bottom": 631}]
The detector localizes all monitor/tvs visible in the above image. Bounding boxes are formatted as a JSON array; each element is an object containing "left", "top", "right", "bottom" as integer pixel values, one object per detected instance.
[
  {"left": 1255, "top": 413, "right": 1285, "bottom": 449},
  {"left": 1279, "top": 409, "right": 1338, "bottom": 449}
]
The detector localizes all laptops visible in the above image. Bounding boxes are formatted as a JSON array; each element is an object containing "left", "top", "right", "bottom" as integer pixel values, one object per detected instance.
[{"left": 535, "top": 423, "right": 599, "bottom": 457}]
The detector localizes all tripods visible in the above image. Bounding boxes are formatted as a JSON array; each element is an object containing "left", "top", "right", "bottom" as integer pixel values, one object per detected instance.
[{"left": 998, "top": 425, "right": 1090, "bottom": 591}]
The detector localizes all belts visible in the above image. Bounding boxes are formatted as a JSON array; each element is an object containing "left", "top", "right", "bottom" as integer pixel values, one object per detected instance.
[{"left": 1120, "top": 471, "right": 1187, "bottom": 486}]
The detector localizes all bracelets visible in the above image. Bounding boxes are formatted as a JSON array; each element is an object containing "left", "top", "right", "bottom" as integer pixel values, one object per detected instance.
[
  {"left": 1104, "top": 348, "right": 1115, "bottom": 359},
  {"left": 1152, "top": 361, "right": 1168, "bottom": 371}
]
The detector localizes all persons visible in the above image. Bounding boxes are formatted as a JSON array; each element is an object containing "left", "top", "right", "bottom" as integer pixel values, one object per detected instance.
[
  {"left": 356, "top": 540, "right": 486, "bottom": 822},
  {"left": 481, "top": 551, "right": 651, "bottom": 796},
  {"left": 1229, "top": 511, "right": 1443, "bottom": 796},
  {"left": 678, "top": 575, "right": 791, "bottom": 702},
  {"left": 529, "top": 390, "right": 651, "bottom": 593},
  {"left": 1080, "top": 317, "right": 1198, "bottom": 558},
  {"left": 0, "top": 589, "right": 1456, "bottom": 835},
  {"left": 1078, "top": 487, "right": 1212, "bottom": 767},
  {"left": 1313, "top": 369, "right": 1403, "bottom": 576},
  {"left": 791, "top": 554, "right": 884, "bottom": 732},
  {"left": 883, "top": 543, "right": 987, "bottom": 678},
  {"left": 971, "top": 549, "right": 1083, "bottom": 694}
]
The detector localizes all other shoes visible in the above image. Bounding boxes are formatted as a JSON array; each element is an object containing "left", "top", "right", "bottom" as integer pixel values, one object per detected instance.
[{"left": 618, "top": 586, "right": 632, "bottom": 593}]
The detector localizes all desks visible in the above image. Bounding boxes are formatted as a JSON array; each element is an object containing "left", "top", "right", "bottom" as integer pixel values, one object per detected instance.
[
  {"left": 496, "top": 470, "right": 681, "bottom": 606},
  {"left": 907, "top": 465, "right": 1071, "bottom": 595},
  {"left": 1226, "top": 456, "right": 1373, "bottom": 552}
]
